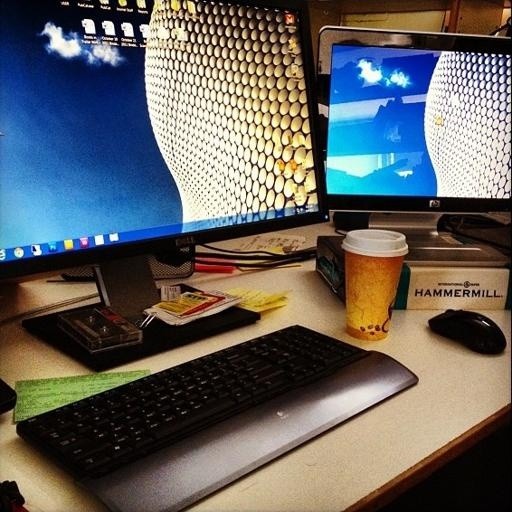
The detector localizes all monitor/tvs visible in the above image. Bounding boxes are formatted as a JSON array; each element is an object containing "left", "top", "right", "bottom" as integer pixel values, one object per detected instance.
[
  {"left": 1, "top": 0, "right": 331, "bottom": 372},
  {"left": 318, "top": 25, "right": 512, "bottom": 234}
]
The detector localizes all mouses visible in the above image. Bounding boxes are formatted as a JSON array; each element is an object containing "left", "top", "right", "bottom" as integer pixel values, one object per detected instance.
[{"left": 428, "top": 309, "right": 507, "bottom": 356}]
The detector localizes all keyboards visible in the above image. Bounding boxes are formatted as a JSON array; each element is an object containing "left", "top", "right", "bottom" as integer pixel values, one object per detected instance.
[{"left": 16, "top": 324, "right": 418, "bottom": 511}]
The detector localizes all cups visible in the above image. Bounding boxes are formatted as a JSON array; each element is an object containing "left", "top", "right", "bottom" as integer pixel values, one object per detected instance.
[{"left": 340, "top": 229, "right": 409, "bottom": 341}]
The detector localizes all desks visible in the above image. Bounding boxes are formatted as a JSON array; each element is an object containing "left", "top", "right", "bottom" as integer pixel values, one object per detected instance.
[{"left": 0, "top": 223, "right": 511, "bottom": 512}]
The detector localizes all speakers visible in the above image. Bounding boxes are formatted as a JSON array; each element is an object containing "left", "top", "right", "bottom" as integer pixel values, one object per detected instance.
[{"left": 60, "top": 245, "right": 195, "bottom": 283}]
